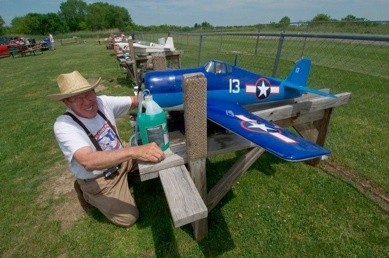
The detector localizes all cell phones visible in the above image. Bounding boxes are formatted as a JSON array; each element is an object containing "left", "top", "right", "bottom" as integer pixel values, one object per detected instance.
[{"left": 105, "top": 167, "right": 119, "bottom": 178}]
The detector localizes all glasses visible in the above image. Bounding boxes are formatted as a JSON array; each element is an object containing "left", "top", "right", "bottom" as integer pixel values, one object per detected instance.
[{"left": 65, "top": 93, "right": 96, "bottom": 105}]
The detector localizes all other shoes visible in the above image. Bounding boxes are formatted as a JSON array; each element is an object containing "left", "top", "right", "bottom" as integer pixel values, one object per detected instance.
[{"left": 74, "top": 180, "right": 93, "bottom": 209}]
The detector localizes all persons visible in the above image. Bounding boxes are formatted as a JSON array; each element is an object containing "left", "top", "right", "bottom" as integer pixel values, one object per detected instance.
[
  {"left": 106, "top": 32, "right": 128, "bottom": 50},
  {"left": 7, "top": 31, "right": 56, "bottom": 59},
  {"left": 46, "top": 69, "right": 166, "bottom": 228}
]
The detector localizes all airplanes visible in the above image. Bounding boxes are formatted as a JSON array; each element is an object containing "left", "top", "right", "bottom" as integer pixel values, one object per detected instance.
[
  {"left": 138, "top": 55, "right": 338, "bottom": 163},
  {"left": 113, "top": 37, "right": 175, "bottom": 53}
]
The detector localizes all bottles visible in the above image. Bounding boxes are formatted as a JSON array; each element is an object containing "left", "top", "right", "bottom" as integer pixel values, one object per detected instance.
[{"left": 137, "top": 95, "right": 170, "bottom": 151}]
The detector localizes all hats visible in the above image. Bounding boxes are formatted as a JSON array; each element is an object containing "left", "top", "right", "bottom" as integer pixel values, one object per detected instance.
[{"left": 46, "top": 70, "right": 101, "bottom": 102}]
